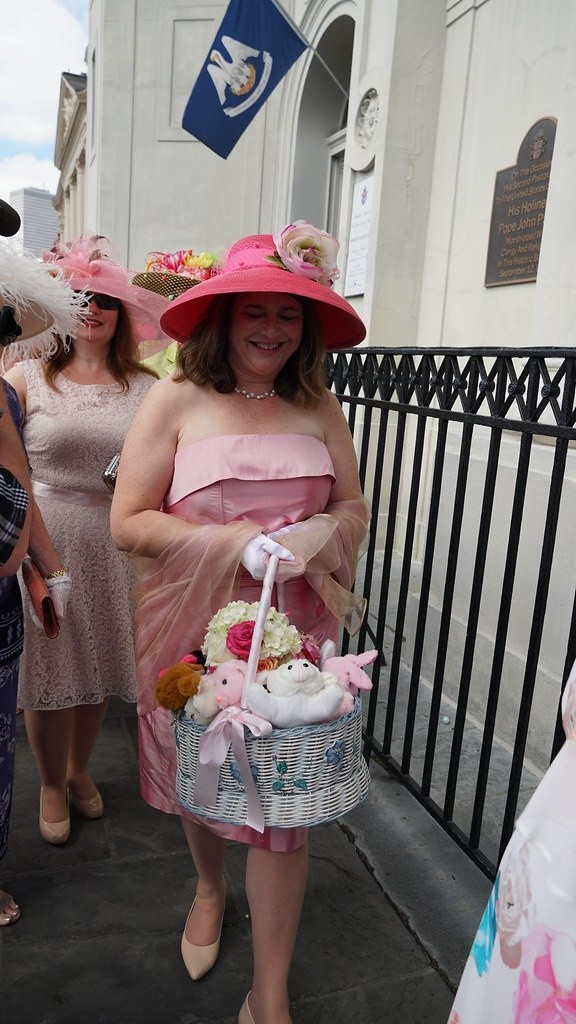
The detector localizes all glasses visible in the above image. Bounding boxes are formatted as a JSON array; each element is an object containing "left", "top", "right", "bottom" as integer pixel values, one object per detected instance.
[{"left": 66, "top": 287, "right": 121, "bottom": 312}]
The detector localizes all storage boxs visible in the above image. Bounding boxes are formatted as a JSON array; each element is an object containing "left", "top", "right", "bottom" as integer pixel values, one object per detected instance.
[{"left": 170, "top": 553, "right": 372, "bottom": 829}]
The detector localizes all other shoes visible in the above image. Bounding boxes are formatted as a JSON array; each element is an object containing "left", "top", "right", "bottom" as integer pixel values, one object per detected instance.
[
  {"left": 180, "top": 876, "right": 228, "bottom": 979},
  {"left": 238, "top": 993, "right": 260, "bottom": 1023},
  {"left": 38, "top": 782, "right": 71, "bottom": 844},
  {"left": 67, "top": 783, "right": 102, "bottom": 817}
]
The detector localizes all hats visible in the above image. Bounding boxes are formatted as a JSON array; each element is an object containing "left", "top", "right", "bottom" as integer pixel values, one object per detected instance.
[
  {"left": 132, "top": 250, "right": 222, "bottom": 299},
  {"left": 36, "top": 236, "right": 175, "bottom": 363},
  {"left": 0, "top": 239, "right": 94, "bottom": 374},
  {"left": 159, "top": 220, "right": 366, "bottom": 352}
]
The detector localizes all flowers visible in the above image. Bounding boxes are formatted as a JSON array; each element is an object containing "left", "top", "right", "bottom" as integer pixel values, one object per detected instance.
[
  {"left": 263, "top": 219, "right": 341, "bottom": 288},
  {"left": 145, "top": 249, "right": 223, "bottom": 282},
  {"left": 179, "top": 600, "right": 320, "bottom": 674}
]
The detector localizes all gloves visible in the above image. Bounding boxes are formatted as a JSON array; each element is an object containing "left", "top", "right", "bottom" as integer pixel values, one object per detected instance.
[
  {"left": 29, "top": 574, "right": 72, "bottom": 631},
  {"left": 238, "top": 533, "right": 295, "bottom": 580},
  {"left": 267, "top": 521, "right": 307, "bottom": 540}
]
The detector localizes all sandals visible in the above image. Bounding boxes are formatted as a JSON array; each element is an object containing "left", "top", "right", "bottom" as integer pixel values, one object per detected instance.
[{"left": 0, "top": 891, "right": 21, "bottom": 925}]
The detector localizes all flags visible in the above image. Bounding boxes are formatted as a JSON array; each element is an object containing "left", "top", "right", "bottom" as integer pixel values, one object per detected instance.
[{"left": 181, "top": 0, "right": 307, "bottom": 161}]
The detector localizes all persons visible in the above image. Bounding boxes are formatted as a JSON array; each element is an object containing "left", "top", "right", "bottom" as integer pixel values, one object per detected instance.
[{"left": 1, "top": 200, "right": 377, "bottom": 1023}]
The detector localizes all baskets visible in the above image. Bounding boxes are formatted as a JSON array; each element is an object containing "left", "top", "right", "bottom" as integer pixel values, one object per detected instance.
[{"left": 169, "top": 558, "right": 370, "bottom": 835}]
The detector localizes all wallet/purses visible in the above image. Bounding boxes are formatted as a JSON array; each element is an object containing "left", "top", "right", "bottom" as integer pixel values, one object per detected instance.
[
  {"left": 102, "top": 453, "right": 122, "bottom": 493},
  {"left": 20, "top": 558, "right": 61, "bottom": 641}
]
